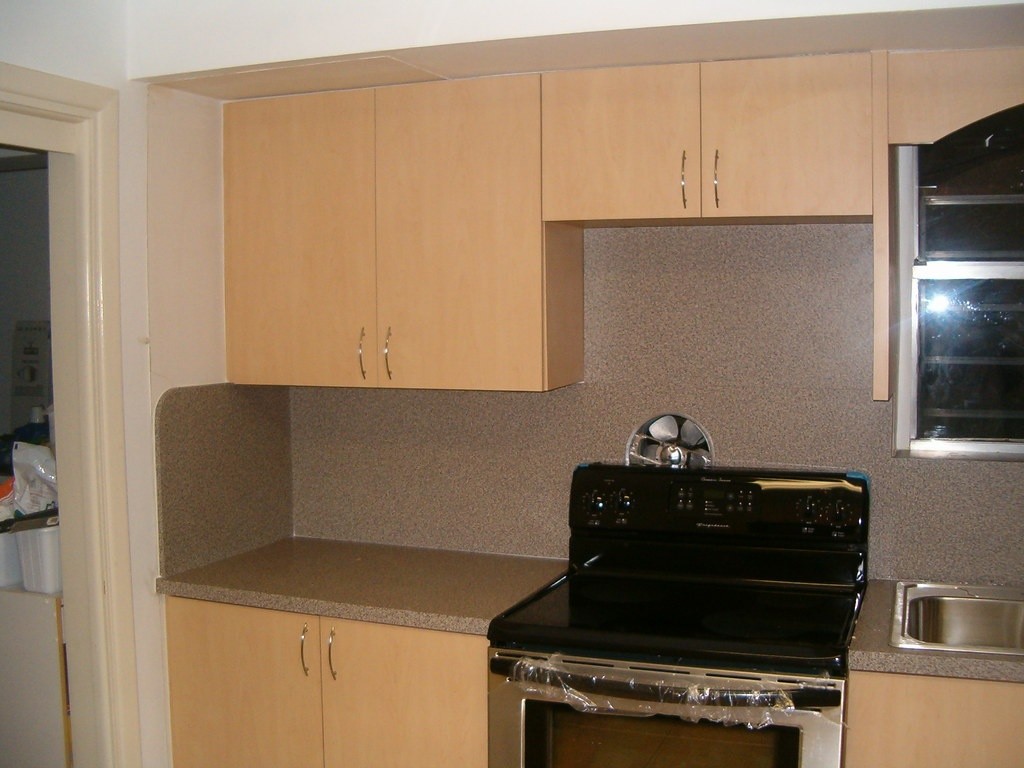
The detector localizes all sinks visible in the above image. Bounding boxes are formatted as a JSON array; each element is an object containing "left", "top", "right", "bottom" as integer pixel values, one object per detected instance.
[{"left": 888, "top": 579, "right": 1024, "bottom": 655}]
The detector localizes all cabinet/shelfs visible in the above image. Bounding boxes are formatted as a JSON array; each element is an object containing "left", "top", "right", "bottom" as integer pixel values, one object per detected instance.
[
  {"left": 223, "top": 73, "right": 586, "bottom": 392},
  {"left": 842, "top": 670, "right": 1024, "bottom": 768},
  {"left": 166, "top": 595, "right": 527, "bottom": 768},
  {"left": 541, "top": 52, "right": 873, "bottom": 225},
  {"left": 0, "top": 581, "right": 73, "bottom": 768}
]
